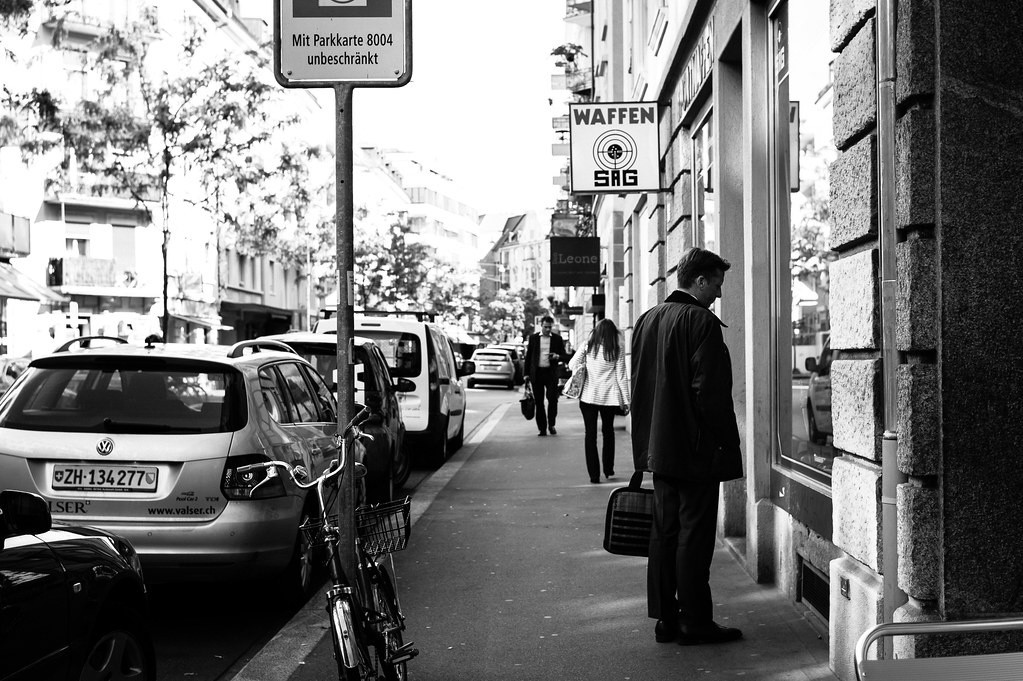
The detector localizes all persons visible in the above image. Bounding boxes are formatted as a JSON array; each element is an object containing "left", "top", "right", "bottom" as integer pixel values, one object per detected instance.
[
  {"left": 568, "top": 319, "right": 631, "bottom": 485},
  {"left": 629, "top": 248, "right": 744, "bottom": 644},
  {"left": 524, "top": 316, "right": 576, "bottom": 438}
]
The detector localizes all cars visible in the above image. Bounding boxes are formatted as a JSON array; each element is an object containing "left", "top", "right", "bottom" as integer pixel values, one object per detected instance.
[
  {"left": 484, "top": 343, "right": 527, "bottom": 386},
  {"left": 805, "top": 335, "right": 834, "bottom": 445},
  {"left": 0, "top": 354, "right": 32, "bottom": 399},
  {"left": 468, "top": 349, "right": 515, "bottom": 390},
  {"left": 253, "top": 335, "right": 411, "bottom": 513},
  {"left": 0, "top": 490, "right": 150, "bottom": 680}
]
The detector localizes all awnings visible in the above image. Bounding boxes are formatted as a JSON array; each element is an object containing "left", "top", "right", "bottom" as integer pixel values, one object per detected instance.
[
  {"left": 0, "top": 261, "right": 70, "bottom": 305},
  {"left": 171, "top": 312, "right": 234, "bottom": 338},
  {"left": 52, "top": 282, "right": 160, "bottom": 304},
  {"left": 445, "top": 323, "right": 480, "bottom": 346}
]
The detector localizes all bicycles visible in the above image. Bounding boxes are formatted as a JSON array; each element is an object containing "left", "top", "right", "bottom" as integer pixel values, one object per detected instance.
[{"left": 236, "top": 406, "right": 419, "bottom": 681}]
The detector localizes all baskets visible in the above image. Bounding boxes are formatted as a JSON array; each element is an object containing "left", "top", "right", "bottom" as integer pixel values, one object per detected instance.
[{"left": 299, "top": 495, "right": 410, "bottom": 565}]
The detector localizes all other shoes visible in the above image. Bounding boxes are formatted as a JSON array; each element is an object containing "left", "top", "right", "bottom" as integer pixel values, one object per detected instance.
[
  {"left": 605, "top": 470, "right": 614, "bottom": 477},
  {"left": 538, "top": 430, "right": 546, "bottom": 436},
  {"left": 591, "top": 478, "right": 600, "bottom": 483},
  {"left": 548, "top": 426, "right": 557, "bottom": 435}
]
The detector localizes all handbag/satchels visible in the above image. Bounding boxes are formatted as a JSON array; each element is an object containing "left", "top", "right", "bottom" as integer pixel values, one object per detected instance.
[
  {"left": 562, "top": 341, "right": 586, "bottom": 400},
  {"left": 604, "top": 469, "right": 658, "bottom": 555},
  {"left": 519, "top": 378, "right": 535, "bottom": 420}
]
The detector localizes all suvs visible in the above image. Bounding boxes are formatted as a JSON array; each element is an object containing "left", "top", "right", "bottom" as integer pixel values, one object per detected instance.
[{"left": 0, "top": 335, "right": 368, "bottom": 612}]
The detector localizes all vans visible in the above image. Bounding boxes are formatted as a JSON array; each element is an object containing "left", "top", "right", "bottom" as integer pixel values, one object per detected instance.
[{"left": 308, "top": 309, "right": 477, "bottom": 469}]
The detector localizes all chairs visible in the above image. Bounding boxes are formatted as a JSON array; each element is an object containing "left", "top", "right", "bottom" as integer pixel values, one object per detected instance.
[{"left": 127, "top": 372, "right": 183, "bottom": 418}]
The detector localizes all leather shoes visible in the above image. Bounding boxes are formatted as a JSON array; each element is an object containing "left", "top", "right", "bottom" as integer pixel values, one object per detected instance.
[
  {"left": 677, "top": 620, "right": 742, "bottom": 647},
  {"left": 655, "top": 619, "right": 678, "bottom": 643}
]
